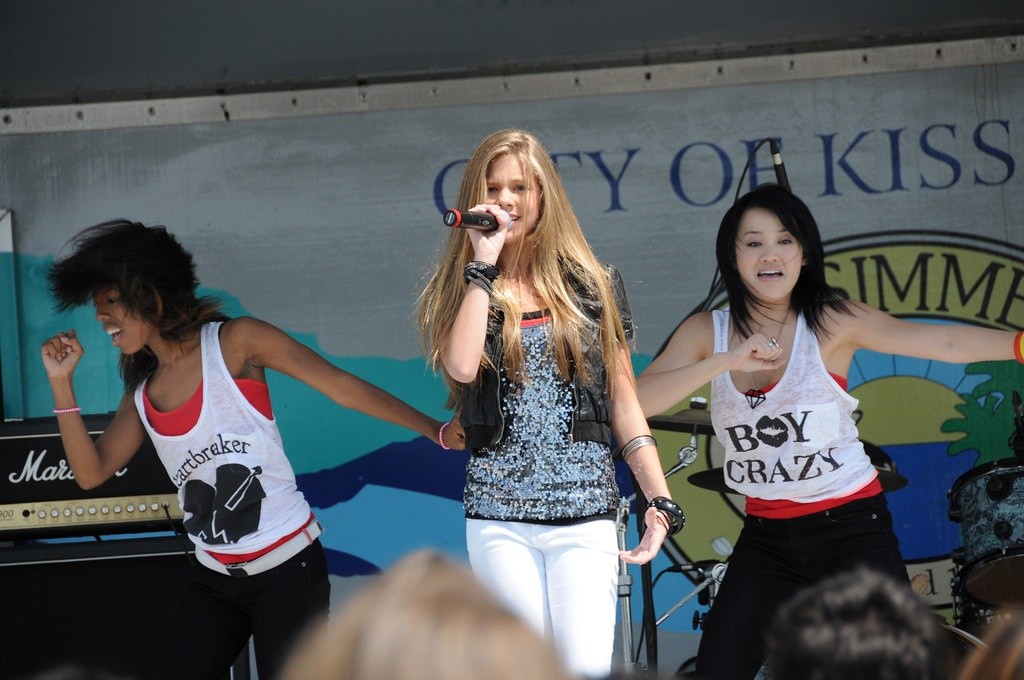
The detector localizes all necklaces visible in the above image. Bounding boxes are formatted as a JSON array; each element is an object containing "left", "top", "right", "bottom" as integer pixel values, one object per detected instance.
[{"left": 738, "top": 304, "right": 792, "bottom": 409}]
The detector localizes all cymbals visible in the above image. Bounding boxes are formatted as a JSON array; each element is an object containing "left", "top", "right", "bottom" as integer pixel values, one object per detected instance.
[
  {"left": 646, "top": 407, "right": 717, "bottom": 436},
  {"left": 688, "top": 465, "right": 909, "bottom": 498}
]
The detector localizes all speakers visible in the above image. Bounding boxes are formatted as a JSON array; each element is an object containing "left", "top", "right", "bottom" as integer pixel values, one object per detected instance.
[{"left": 0, "top": 534, "right": 251, "bottom": 680}]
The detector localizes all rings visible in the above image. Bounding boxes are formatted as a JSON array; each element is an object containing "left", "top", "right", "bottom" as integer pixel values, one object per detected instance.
[{"left": 768, "top": 336, "right": 780, "bottom": 349}]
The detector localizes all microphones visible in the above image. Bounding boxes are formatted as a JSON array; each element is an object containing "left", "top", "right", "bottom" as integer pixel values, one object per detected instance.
[
  {"left": 668, "top": 559, "right": 720, "bottom": 573},
  {"left": 443, "top": 209, "right": 512, "bottom": 232}
]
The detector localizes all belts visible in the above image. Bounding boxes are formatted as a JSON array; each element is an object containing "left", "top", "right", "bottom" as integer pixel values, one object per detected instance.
[{"left": 194, "top": 519, "right": 325, "bottom": 576}]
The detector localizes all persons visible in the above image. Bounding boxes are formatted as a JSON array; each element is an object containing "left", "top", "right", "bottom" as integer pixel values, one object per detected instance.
[
  {"left": 765, "top": 568, "right": 953, "bottom": 680},
  {"left": 416, "top": 130, "right": 673, "bottom": 680},
  {"left": 277, "top": 548, "right": 583, "bottom": 680},
  {"left": 635, "top": 182, "right": 1024, "bottom": 680},
  {"left": 40, "top": 220, "right": 467, "bottom": 680}
]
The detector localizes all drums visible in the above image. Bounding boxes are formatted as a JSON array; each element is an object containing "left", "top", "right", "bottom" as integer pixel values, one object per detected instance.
[{"left": 948, "top": 456, "right": 1024, "bottom": 610}]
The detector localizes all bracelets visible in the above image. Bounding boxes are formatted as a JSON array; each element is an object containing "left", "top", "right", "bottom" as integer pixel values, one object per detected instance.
[
  {"left": 439, "top": 423, "right": 451, "bottom": 450},
  {"left": 1013, "top": 331, "right": 1024, "bottom": 364},
  {"left": 648, "top": 494, "right": 687, "bottom": 536},
  {"left": 53, "top": 407, "right": 81, "bottom": 414},
  {"left": 463, "top": 260, "right": 502, "bottom": 297}
]
